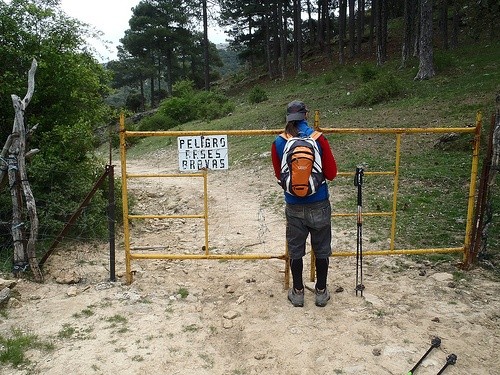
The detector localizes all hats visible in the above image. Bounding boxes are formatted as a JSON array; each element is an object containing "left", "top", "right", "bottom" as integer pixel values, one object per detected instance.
[{"left": 287, "top": 100, "right": 306, "bottom": 121}]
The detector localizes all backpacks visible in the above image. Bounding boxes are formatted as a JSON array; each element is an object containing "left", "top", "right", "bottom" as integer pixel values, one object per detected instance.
[{"left": 277, "top": 131, "right": 326, "bottom": 197}]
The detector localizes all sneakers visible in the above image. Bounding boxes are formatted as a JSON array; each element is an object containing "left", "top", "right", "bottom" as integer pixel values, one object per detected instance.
[
  {"left": 288, "top": 288, "right": 304, "bottom": 307},
  {"left": 315, "top": 286, "right": 330, "bottom": 307}
]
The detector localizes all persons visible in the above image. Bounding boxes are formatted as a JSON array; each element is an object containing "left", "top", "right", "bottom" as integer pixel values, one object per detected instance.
[{"left": 271, "top": 100, "right": 337, "bottom": 307}]
